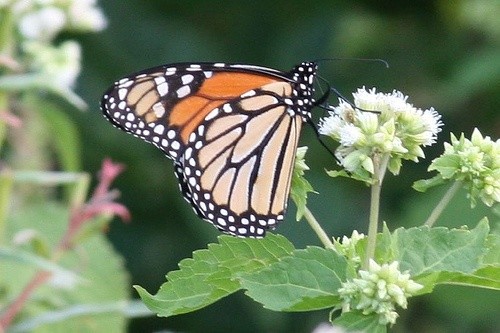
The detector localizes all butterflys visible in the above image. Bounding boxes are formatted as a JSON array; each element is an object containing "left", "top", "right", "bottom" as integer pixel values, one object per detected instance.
[{"left": 99, "top": 55, "right": 390, "bottom": 240}]
